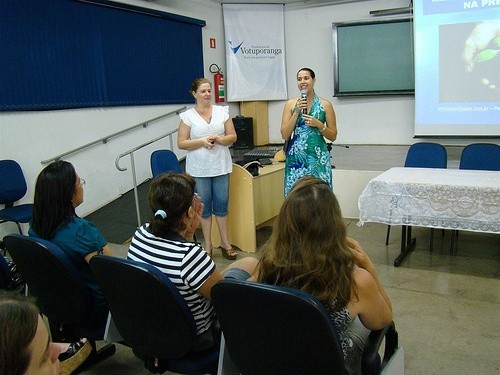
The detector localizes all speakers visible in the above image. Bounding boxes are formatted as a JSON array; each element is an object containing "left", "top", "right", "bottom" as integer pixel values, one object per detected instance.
[{"left": 232, "top": 116, "right": 254, "bottom": 149}]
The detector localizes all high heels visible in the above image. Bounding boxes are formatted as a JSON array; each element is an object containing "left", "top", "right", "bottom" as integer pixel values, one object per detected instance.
[
  {"left": 206, "top": 249, "right": 213, "bottom": 258},
  {"left": 220, "top": 245, "right": 237, "bottom": 260}
]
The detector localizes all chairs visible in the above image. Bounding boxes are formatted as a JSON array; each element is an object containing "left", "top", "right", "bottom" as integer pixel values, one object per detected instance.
[
  {"left": 150, "top": 150, "right": 197, "bottom": 242},
  {"left": 450, "top": 144, "right": 500, "bottom": 255},
  {"left": 3, "top": 234, "right": 404, "bottom": 375},
  {"left": 386, "top": 142, "right": 447, "bottom": 251},
  {"left": 0, "top": 160, "right": 34, "bottom": 234}
]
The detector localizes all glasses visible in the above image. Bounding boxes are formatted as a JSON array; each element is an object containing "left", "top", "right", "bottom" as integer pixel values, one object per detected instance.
[
  {"left": 190, "top": 193, "right": 197, "bottom": 207},
  {"left": 75, "top": 179, "right": 85, "bottom": 189}
]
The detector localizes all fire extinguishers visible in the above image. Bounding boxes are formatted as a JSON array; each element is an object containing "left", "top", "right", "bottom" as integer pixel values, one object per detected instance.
[{"left": 214, "top": 68, "right": 226, "bottom": 103}]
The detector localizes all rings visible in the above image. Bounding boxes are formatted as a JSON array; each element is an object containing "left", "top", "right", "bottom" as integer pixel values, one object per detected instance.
[{"left": 307, "top": 120, "right": 310, "bottom": 124}]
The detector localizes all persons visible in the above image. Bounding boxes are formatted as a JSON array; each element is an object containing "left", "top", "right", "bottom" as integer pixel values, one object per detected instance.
[
  {"left": 247, "top": 174, "right": 393, "bottom": 375},
  {"left": 177, "top": 77, "right": 238, "bottom": 261},
  {"left": 0, "top": 251, "right": 87, "bottom": 375},
  {"left": 125, "top": 173, "right": 258, "bottom": 353},
  {"left": 27, "top": 161, "right": 113, "bottom": 262},
  {"left": 461, "top": 21, "right": 500, "bottom": 91},
  {"left": 280, "top": 68, "right": 337, "bottom": 199}
]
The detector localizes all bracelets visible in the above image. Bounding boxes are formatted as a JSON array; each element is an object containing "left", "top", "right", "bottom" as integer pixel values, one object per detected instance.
[{"left": 319, "top": 124, "right": 327, "bottom": 132}]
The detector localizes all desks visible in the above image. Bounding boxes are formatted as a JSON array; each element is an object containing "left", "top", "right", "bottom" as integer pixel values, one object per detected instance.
[
  {"left": 211, "top": 150, "right": 286, "bottom": 253},
  {"left": 356, "top": 167, "right": 500, "bottom": 267}
]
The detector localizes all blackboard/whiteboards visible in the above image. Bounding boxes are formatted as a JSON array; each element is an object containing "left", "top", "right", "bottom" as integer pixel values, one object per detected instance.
[{"left": 332, "top": 16, "right": 415, "bottom": 96}]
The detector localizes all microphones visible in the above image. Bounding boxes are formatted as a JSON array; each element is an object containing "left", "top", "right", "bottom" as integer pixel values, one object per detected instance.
[{"left": 301, "top": 90, "right": 307, "bottom": 114}]
